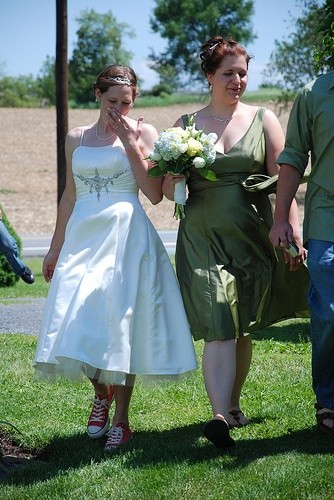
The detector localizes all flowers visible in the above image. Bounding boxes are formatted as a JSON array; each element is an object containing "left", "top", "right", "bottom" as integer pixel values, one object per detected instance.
[{"left": 140, "top": 112, "right": 219, "bottom": 220}]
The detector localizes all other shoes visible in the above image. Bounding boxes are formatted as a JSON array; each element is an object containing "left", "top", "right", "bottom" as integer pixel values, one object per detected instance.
[
  {"left": 19, "top": 265, "right": 35, "bottom": 284},
  {"left": 227, "top": 409, "right": 244, "bottom": 428},
  {"left": 203, "top": 418, "right": 235, "bottom": 451},
  {"left": 316, "top": 408, "right": 334, "bottom": 435}
]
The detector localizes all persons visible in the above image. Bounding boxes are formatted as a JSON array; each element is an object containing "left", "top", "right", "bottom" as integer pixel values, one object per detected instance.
[
  {"left": 33, "top": 64, "right": 198, "bottom": 453},
  {"left": 162, "top": 35, "right": 305, "bottom": 450},
  {"left": 268, "top": 70, "right": 334, "bottom": 431}
]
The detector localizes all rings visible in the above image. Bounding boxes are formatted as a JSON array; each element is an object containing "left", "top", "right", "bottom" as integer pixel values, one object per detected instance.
[{"left": 115, "top": 125, "right": 118, "bottom": 130}]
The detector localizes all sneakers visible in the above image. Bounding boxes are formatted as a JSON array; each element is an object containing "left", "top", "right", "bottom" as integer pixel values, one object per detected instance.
[
  {"left": 87, "top": 384, "right": 115, "bottom": 439},
  {"left": 104, "top": 422, "right": 133, "bottom": 450}
]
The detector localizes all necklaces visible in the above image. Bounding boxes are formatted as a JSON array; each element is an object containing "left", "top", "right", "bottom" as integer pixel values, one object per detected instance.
[
  {"left": 214, "top": 116, "right": 234, "bottom": 124},
  {"left": 96, "top": 126, "right": 116, "bottom": 141}
]
